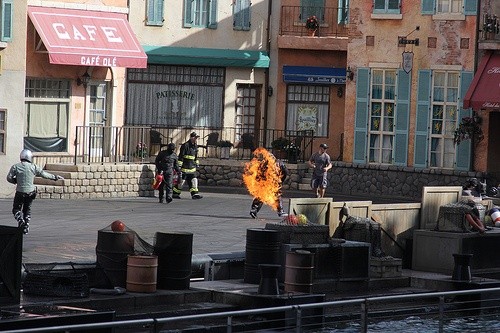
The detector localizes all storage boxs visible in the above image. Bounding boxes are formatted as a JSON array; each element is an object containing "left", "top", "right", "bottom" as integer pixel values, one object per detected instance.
[
  {"left": 460, "top": 199, "right": 492, "bottom": 225},
  {"left": 436, "top": 204, "right": 485, "bottom": 232}
]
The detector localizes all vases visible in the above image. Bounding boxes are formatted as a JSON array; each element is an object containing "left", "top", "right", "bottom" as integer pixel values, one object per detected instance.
[
  {"left": 308, "top": 28, "right": 316, "bottom": 36},
  {"left": 288, "top": 151, "right": 299, "bottom": 164}
]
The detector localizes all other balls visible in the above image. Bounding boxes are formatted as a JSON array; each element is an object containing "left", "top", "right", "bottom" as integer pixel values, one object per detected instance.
[
  {"left": 296, "top": 213, "right": 307, "bottom": 225},
  {"left": 112, "top": 221, "right": 124, "bottom": 232},
  {"left": 287, "top": 215, "right": 298, "bottom": 225}
]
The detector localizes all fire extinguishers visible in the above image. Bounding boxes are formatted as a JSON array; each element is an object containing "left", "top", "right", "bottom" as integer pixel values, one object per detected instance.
[
  {"left": 151, "top": 171, "right": 164, "bottom": 190},
  {"left": 173, "top": 168, "right": 178, "bottom": 185}
]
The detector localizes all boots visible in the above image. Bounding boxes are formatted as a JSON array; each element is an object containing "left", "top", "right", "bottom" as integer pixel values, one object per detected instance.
[
  {"left": 23, "top": 214, "right": 30, "bottom": 234},
  {"left": 14, "top": 210, "right": 25, "bottom": 229}
]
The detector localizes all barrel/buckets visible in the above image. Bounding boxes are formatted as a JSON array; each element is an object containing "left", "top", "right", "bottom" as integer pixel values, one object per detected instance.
[
  {"left": 284, "top": 250, "right": 314, "bottom": 294},
  {"left": 126, "top": 255, "right": 158, "bottom": 293},
  {"left": 95, "top": 230, "right": 135, "bottom": 288},
  {"left": 244, "top": 227, "right": 281, "bottom": 284},
  {"left": 153, "top": 231, "right": 194, "bottom": 290}
]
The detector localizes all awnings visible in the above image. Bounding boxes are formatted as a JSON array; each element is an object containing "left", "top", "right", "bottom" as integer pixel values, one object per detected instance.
[
  {"left": 28, "top": 5, "right": 148, "bottom": 69},
  {"left": 463, "top": 51, "right": 500, "bottom": 110}
]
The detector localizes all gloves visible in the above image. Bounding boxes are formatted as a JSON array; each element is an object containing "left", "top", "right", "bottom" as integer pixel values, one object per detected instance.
[{"left": 55, "top": 175, "right": 62, "bottom": 181}]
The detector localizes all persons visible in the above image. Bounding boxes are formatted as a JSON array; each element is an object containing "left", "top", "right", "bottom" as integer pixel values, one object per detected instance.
[
  {"left": 172, "top": 132, "right": 203, "bottom": 200},
  {"left": 155, "top": 143, "right": 182, "bottom": 203},
  {"left": 7, "top": 150, "right": 64, "bottom": 234},
  {"left": 250, "top": 154, "right": 288, "bottom": 218},
  {"left": 307, "top": 143, "right": 333, "bottom": 198}
]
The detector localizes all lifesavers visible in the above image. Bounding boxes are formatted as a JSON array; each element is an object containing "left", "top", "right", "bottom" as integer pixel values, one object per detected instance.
[{"left": 467, "top": 212, "right": 485, "bottom": 232}]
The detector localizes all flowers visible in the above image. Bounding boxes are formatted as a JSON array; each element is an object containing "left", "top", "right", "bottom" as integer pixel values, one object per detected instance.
[
  {"left": 305, "top": 16, "right": 320, "bottom": 30},
  {"left": 453, "top": 117, "right": 484, "bottom": 147},
  {"left": 283, "top": 142, "right": 301, "bottom": 156}
]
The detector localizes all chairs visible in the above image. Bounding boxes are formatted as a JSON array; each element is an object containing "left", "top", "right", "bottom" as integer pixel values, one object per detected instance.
[
  {"left": 147, "top": 128, "right": 176, "bottom": 156},
  {"left": 293, "top": 136, "right": 302, "bottom": 161},
  {"left": 197, "top": 132, "right": 219, "bottom": 157}
]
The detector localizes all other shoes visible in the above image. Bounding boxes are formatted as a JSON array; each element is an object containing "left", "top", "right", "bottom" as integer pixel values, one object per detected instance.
[
  {"left": 159, "top": 200, "right": 163, "bottom": 203},
  {"left": 172, "top": 195, "right": 180, "bottom": 199},
  {"left": 166, "top": 197, "right": 172, "bottom": 203},
  {"left": 249, "top": 211, "right": 257, "bottom": 219},
  {"left": 191, "top": 194, "right": 203, "bottom": 200},
  {"left": 278, "top": 212, "right": 288, "bottom": 216}
]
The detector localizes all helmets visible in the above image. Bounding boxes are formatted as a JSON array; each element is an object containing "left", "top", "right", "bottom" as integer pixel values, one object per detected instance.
[{"left": 20, "top": 149, "right": 32, "bottom": 161}]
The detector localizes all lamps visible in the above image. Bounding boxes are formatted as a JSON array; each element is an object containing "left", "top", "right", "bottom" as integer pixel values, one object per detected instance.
[
  {"left": 268, "top": 86, "right": 272, "bottom": 95},
  {"left": 76, "top": 72, "right": 91, "bottom": 88},
  {"left": 337, "top": 86, "right": 343, "bottom": 96},
  {"left": 346, "top": 66, "right": 354, "bottom": 81},
  {"left": 474, "top": 112, "right": 480, "bottom": 123}
]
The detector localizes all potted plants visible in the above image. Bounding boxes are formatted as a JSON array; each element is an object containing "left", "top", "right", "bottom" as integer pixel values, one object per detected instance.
[
  {"left": 216, "top": 140, "right": 234, "bottom": 160},
  {"left": 271, "top": 137, "right": 291, "bottom": 160}
]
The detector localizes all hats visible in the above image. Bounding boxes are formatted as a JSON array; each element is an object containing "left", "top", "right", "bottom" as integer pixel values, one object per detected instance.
[
  {"left": 168, "top": 142, "right": 176, "bottom": 149},
  {"left": 320, "top": 143, "right": 328, "bottom": 148},
  {"left": 190, "top": 132, "right": 200, "bottom": 138}
]
